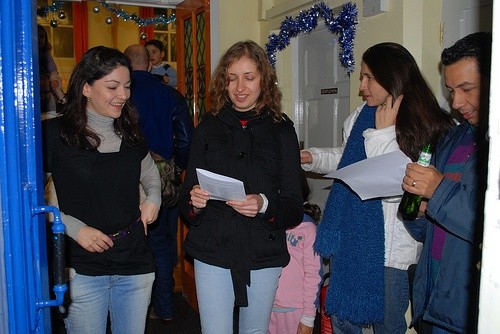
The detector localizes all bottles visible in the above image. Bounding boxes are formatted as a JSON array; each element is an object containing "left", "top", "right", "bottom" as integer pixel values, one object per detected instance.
[{"left": 399, "top": 143, "right": 435, "bottom": 219}]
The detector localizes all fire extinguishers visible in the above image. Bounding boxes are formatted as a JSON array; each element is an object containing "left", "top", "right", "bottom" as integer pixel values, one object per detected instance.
[{"left": 321, "top": 272, "right": 333, "bottom": 334}]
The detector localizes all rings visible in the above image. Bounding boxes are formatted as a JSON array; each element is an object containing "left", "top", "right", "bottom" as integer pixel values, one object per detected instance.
[{"left": 411, "top": 181, "right": 416, "bottom": 186}]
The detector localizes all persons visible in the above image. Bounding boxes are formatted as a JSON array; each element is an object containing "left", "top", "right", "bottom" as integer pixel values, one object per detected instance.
[
  {"left": 299, "top": 43, "right": 449, "bottom": 334},
  {"left": 178, "top": 40, "right": 304, "bottom": 334},
  {"left": 37, "top": 25, "right": 194, "bottom": 320},
  {"left": 403, "top": 32, "right": 492, "bottom": 334},
  {"left": 40, "top": 45, "right": 162, "bottom": 334},
  {"left": 268, "top": 180, "right": 321, "bottom": 334}
]
